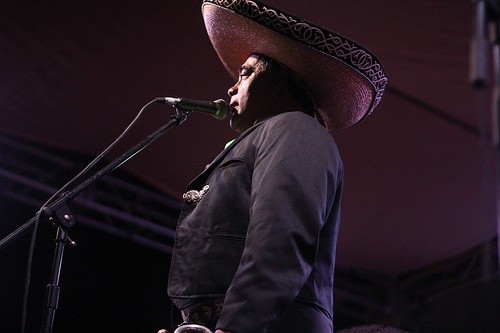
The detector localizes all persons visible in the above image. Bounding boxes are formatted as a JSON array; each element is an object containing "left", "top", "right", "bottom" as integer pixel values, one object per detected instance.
[{"left": 168, "top": 39, "right": 345, "bottom": 333}]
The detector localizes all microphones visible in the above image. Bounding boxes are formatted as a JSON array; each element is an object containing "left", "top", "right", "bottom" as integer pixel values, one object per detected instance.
[{"left": 157, "top": 97, "right": 231, "bottom": 120}]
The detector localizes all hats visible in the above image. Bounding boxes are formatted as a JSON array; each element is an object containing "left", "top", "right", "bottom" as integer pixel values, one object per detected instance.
[{"left": 201, "top": 0, "right": 388, "bottom": 133}]
